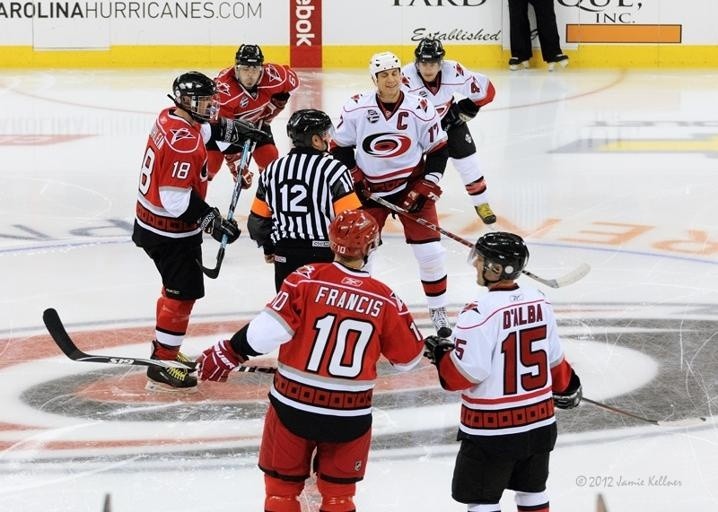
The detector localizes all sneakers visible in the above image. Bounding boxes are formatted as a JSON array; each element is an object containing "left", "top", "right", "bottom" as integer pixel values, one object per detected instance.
[
  {"left": 145, "top": 356, "right": 197, "bottom": 387},
  {"left": 547, "top": 55, "right": 568, "bottom": 62},
  {"left": 475, "top": 203, "right": 496, "bottom": 224},
  {"left": 430, "top": 307, "right": 452, "bottom": 338},
  {"left": 175, "top": 352, "right": 196, "bottom": 373},
  {"left": 509, "top": 57, "right": 529, "bottom": 65}
]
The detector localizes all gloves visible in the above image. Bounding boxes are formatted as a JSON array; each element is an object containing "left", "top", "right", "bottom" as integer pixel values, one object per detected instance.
[
  {"left": 351, "top": 166, "right": 371, "bottom": 200},
  {"left": 403, "top": 180, "right": 442, "bottom": 216},
  {"left": 200, "top": 207, "right": 241, "bottom": 244},
  {"left": 195, "top": 340, "right": 249, "bottom": 382},
  {"left": 221, "top": 118, "right": 266, "bottom": 148},
  {"left": 446, "top": 98, "right": 479, "bottom": 126},
  {"left": 423, "top": 335, "right": 454, "bottom": 364},
  {"left": 226, "top": 158, "right": 253, "bottom": 189}
]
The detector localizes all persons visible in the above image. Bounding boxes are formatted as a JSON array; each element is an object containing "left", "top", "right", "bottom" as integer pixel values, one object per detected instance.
[
  {"left": 134, "top": 70, "right": 274, "bottom": 388},
  {"left": 199, "top": 43, "right": 299, "bottom": 181},
  {"left": 192, "top": 207, "right": 425, "bottom": 512},
  {"left": 247, "top": 108, "right": 362, "bottom": 295},
  {"left": 397, "top": 39, "right": 497, "bottom": 225},
  {"left": 423, "top": 231, "right": 582, "bottom": 512},
  {"left": 323, "top": 51, "right": 452, "bottom": 338},
  {"left": 507, "top": 0, "right": 568, "bottom": 73}
]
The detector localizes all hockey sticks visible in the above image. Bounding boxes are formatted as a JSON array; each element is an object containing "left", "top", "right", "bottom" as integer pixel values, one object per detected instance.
[
  {"left": 195, "top": 138, "right": 250, "bottom": 279},
  {"left": 436, "top": 328, "right": 661, "bottom": 425},
  {"left": 42, "top": 308, "right": 277, "bottom": 372},
  {"left": 370, "top": 191, "right": 590, "bottom": 288}
]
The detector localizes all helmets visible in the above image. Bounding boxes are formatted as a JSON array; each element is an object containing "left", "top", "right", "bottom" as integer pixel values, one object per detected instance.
[
  {"left": 172, "top": 71, "right": 219, "bottom": 123},
  {"left": 468, "top": 232, "right": 529, "bottom": 279},
  {"left": 287, "top": 109, "right": 335, "bottom": 139},
  {"left": 329, "top": 210, "right": 379, "bottom": 258},
  {"left": 414, "top": 38, "right": 445, "bottom": 63},
  {"left": 235, "top": 44, "right": 264, "bottom": 68},
  {"left": 369, "top": 52, "right": 401, "bottom": 84}
]
